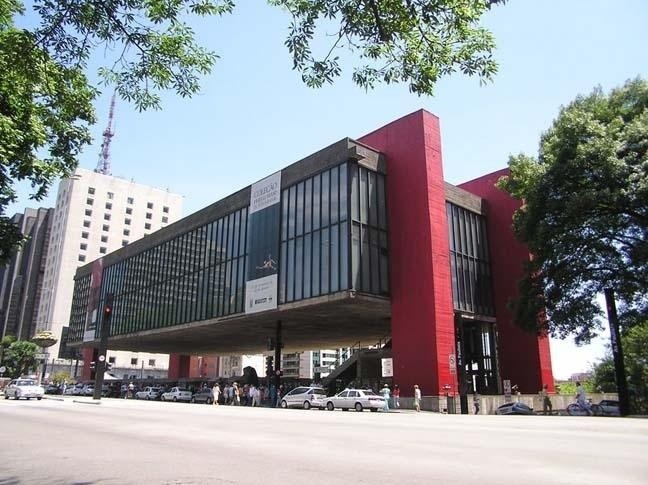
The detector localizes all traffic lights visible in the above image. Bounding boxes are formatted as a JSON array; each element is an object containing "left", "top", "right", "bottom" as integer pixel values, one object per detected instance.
[{"left": 104, "top": 306, "right": 110, "bottom": 321}]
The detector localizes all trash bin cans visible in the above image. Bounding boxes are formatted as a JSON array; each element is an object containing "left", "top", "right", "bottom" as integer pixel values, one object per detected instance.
[{"left": 447, "top": 396, "right": 456, "bottom": 414}]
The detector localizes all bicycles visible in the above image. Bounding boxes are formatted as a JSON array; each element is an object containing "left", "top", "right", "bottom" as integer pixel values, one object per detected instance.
[{"left": 567, "top": 395, "right": 604, "bottom": 416}]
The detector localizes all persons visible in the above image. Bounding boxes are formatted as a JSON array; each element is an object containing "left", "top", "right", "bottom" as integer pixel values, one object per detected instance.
[
  {"left": 379, "top": 384, "right": 391, "bottom": 412},
  {"left": 391, "top": 384, "right": 401, "bottom": 409},
  {"left": 473, "top": 391, "right": 480, "bottom": 415},
  {"left": 413, "top": 384, "right": 422, "bottom": 412},
  {"left": 212, "top": 381, "right": 286, "bottom": 407},
  {"left": 575, "top": 381, "right": 586, "bottom": 411},
  {"left": 541, "top": 383, "right": 552, "bottom": 416}
]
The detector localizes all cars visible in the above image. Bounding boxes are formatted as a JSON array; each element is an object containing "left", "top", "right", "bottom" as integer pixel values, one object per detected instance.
[
  {"left": 62, "top": 384, "right": 220, "bottom": 404},
  {"left": 324, "top": 388, "right": 386, "bottom": 412},
  {"left": 494, "top": 401, "right": 538, "bottom": 416},
  {"left": 279, "top": 386, "right": 328, "bottom": 410},
  {"left": 3, "top": 378, "right": 46, "bottom": 401},
  {"left": 597, "top": 400, "right": 620, "bottom": 416}
]
